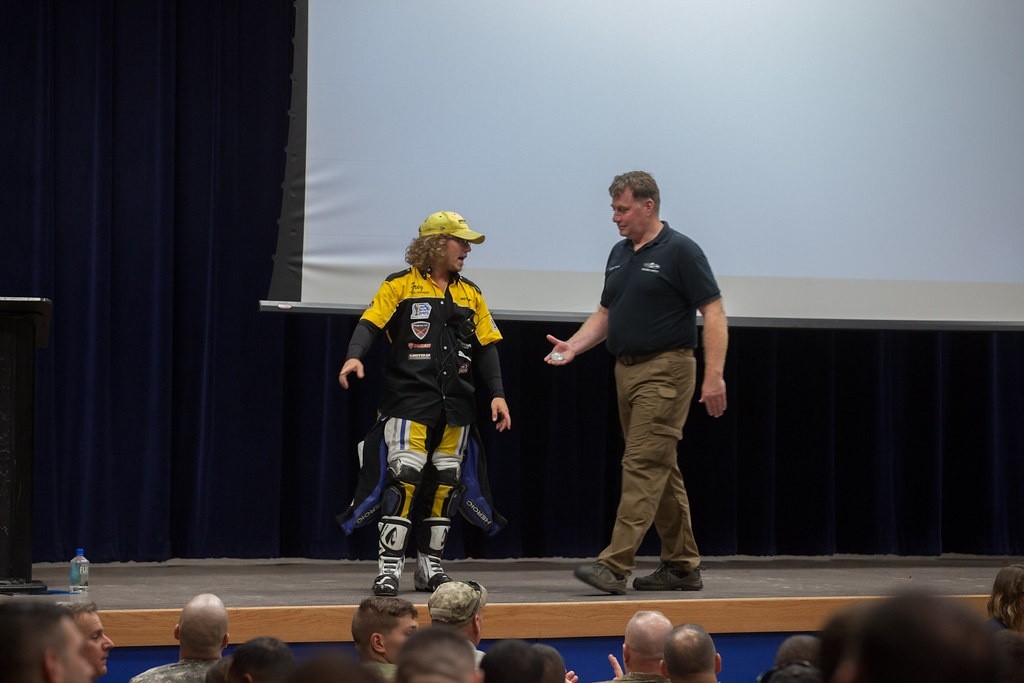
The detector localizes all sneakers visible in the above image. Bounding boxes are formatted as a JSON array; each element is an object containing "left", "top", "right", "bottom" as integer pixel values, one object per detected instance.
[
  {"left": 632, "top": 560, "right": 704, "bottom": 592},
  {"left": 372, "top": 515, "right": 412, "bottom": 596},
  {"left": 413, "top": 516, "right": 454, "bottom": 594},
  {"left": 573, "top": 562, "right": 628, "bottom": 596}
]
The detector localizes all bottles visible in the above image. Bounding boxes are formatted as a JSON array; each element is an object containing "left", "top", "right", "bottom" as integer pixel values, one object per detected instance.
[{"left": 70, "top": 548, "right": 90, "bottom": 594}]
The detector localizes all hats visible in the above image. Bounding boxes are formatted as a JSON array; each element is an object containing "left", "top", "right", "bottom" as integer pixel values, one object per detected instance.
[
  {"left": 427, "top": 580, "right": 489, "bottom": 627},
  {"left": 418, "top": 211, "right": 486, "bottom": 244}
]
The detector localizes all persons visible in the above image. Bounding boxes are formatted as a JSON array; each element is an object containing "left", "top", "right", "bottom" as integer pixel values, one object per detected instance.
[
  {"left": 593, "top": 610, "right": 722, "bottom": 683},
  {"left": 395, "top": 625, "right": 578, "bottom": 683},
  {"left": 0, "top": 597, "right": 113, "bottom": 683},
  {"left": 544, "top": 171, "right": 728, "bottom": 595},
  {"left": 351, "top": 580, "right": 488, "bottom": 683},
  {"left": 129, "top": 593, "right": 230, "bottom": 683},
  {"left": 205, "top": 635, "right": 391, "bottom": 683},
  {"left": 339, "top": 210, "right": 511, "bottom": 596},
  {"left": 760, "top": 564, "right": 1024, "bottom": 683}
]
